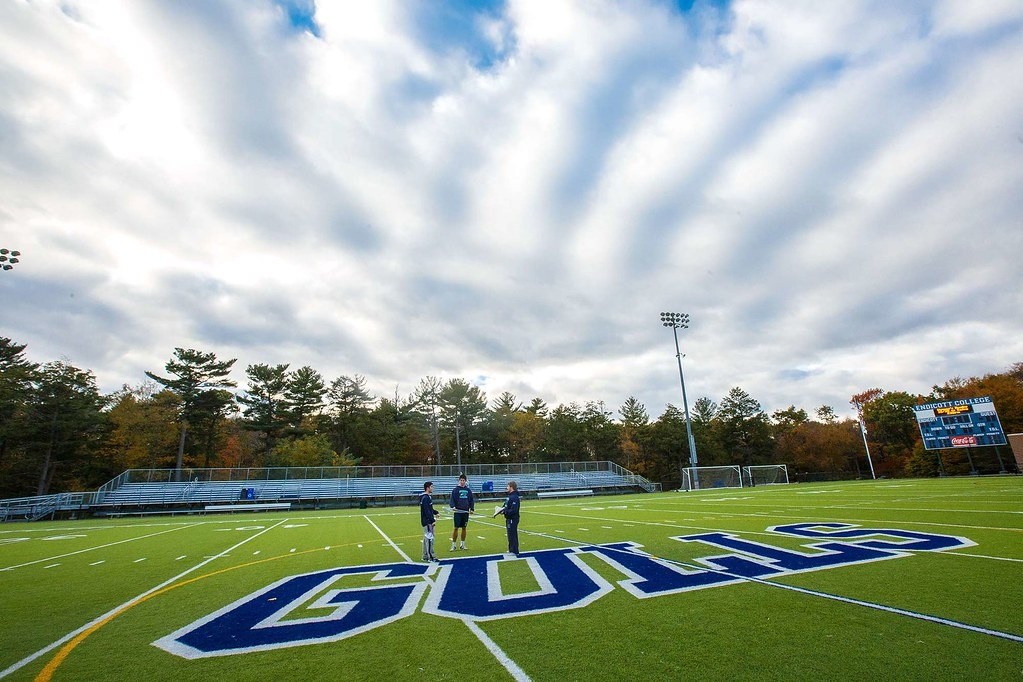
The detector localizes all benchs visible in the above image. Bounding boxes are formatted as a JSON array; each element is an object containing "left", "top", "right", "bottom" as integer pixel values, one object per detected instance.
[{"left": 99, "top": 470, "right": 638, "bottom": 516}]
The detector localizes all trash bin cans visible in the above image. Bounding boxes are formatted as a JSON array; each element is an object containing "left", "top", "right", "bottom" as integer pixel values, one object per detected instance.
[
  {"left": 713, "top": 479, "right": 724, "bottom": 487},
  {"left": 360, "top": 499, "right": 367, "bottom": 509},
  {"left": 240, "top": 487, "right": 255, "bottom": 500},
  {"left": 482, "top": 481, "right": 494, "bottom": 491}
]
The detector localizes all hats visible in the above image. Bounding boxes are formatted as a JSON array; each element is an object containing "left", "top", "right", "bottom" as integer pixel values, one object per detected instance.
[{"left": 492, "top": 505, "right": 505, "bottom": 517}]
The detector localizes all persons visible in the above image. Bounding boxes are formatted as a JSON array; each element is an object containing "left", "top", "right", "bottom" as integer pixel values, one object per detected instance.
[
  {"left": 418, "top": 482, "right": 440, "bottom": 562},
  {"left": 501, "top": 481, "right": 520, "bottom": 556},
  {"left": 448, "top": 474, "right": 474, "bottom": 552}
]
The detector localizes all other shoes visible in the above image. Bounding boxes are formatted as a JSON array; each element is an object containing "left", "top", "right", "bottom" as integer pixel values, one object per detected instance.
[
  {"left": 420, "top": 557, "right": 428, "bottom": 561},
  {"left": 508, "top": 552, "right": 519, "bottom": 556},
  {"left": 427, "top": 556, "right": 439, "bottom": 562},
  {"left": 449, "top": 546, "right": 456, "bottom": 551},
  {"left": 459, "top": 545, "right": 468, "bottom": 549}
]
